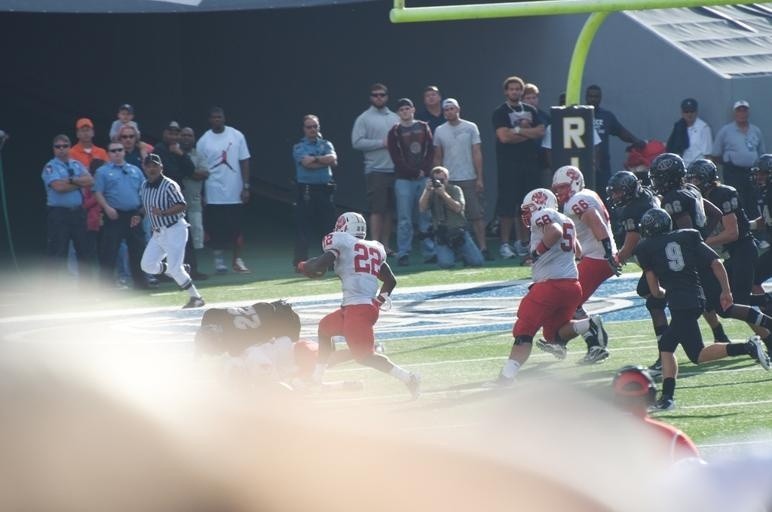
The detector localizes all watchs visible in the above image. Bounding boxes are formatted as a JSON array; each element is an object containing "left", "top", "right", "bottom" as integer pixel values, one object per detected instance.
[
  {"left": 241, "top": 183, "right": 254, "bottom": 191},
  {"left": 314, "top": 154, "right": 322, "bottom": 166},
  {"left": 514, "top": 126, "right": 521, "bottom": 137}
]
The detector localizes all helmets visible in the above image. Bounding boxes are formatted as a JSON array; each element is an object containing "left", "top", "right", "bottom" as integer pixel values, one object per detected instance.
[
  {"left": 638, "top": 208, "right": 672, "bottom": 239},
  {"left": 193, "top": 325, "right": 225, "bottom": 356},
  {"left": 335, "top": 211, "right": 366, "bottom": 240},
  {"left": 684, "top": 162, "right": 719, "bottom": 194},
  {"left": 750, "top": 154, "right": 771, "bottom": 189},
  {"left": 609, "top": 365, "right": 658, "bottom": 410},
  {"left": 552, "top": 166, "right": 585, "bottom": 202},
  {"left": 521, "top": 188, "right": 560, "bottom": 228},
  {"left": 647, "top": 153, "right": 685, "bottom": 196},
  {"left": 293, "top": 340, "right": 319, "bottom": 372},
  {"left": 606, "top": 171, "right": 644, "bottom": 211}
]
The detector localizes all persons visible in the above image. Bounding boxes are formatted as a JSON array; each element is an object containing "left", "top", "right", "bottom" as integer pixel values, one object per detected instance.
[
  {"left": 295, "top": 213, "right": 423, "bottom": 402},
  {"left": 109, "top": 103, "right": 148, "bottom": 165},
  {"left": 431, "top": 99, "right": 493, "bottom": 264},
  {"left": 386, "top": 99, "right": 434, "bottom": 267},
  {"left": 68, "top": 118, "right": 110, "bottom": 172},
  {"left": 608, "top": 171, "right": 676, "bottom": 375},
  {"left": 194, "top": 106, "right": 250, "bottom": 275},
  {"left": 416, "top": 85, "right": 446, "bottom": 132},
  {"left": 41, "top": 134, "right": 95, "bottom": 278},
  {"left": 713, "top": 100, "right": 767, "bottom": 205},
  {"left": 115, "top": 124, "right": 145, "bottom": 168},
  {"left": 542, "top": 94, "right": 602, "bottom": 184},
  {"left": 418, "top": 167, "right": 487, "bottom": 271},
  {"left": 748, "top": 154, "right": 772, "bottom": 313},
  {"left": 130, "top": 154, "right": 205, "bottom": 309},
  {"left": 492, "top": 77, "right": 546, "bottom": 261},
  {"left": 351, "top": 82, "right": 402, "bottom": 257},
  {"left": 583, "top": 85, "right": 646, "bottom": 186},
  {"left": 687, "top": 158, "right": 771, "bottom": 315},
  {"left": 91, "top": 141, "right": 161, "bottom": 290},
  {"left": 497, "top": 189, "right": 607, "bottom": 384},
  {"left": 179, "top": 126, "right": 208, "bottom": 281},
  {"left": 534, "top": 166, "right": 621, "bottom": 366},
  {"left": 292, "top": 115, "right": 339, "bottom": 266},
  {"left": 646, "top": 152, "right": 772, "bottom": 343},
  {"left": 521, "top": 84, "right": 552, "bottom": 189},
  {"left": 663, "top": 97, "right": 713, "bottom": 170},
  {"left": 632, "top": 208, "right": 770, "bottom": 407},
  {"left": 605, "top": 363, "right": 699, "bottom": 461},
  {"left": 150, "top": 121, "right": 196, "bottom": 182}
]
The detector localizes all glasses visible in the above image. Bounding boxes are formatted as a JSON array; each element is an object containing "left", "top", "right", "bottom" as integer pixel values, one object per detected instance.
[
  {"left": 682, "top": 110, "right": 696, "bottom": 113},
  {"left": 121, "top": 134, "right": 136, "bottom": 139},
  {"left": 304, "top": 125, "right": 320, "bottom": 129},
  {"left": 109, "top": 148, "right": 123, "bottom": 152},
  {"left": 54, "top": 144, "right": 70, "bottom": 149},
  {"left": 370, "top": 93, "right": 387, "bottom": 97}
]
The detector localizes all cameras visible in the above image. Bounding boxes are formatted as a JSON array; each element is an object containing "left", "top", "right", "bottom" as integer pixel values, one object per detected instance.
[{"left": 432, "top": 179, "right": 443, "bottom": 188}]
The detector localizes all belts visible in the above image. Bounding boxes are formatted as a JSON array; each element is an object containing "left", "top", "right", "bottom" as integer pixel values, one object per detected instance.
[
  {"left": 723, "top": 161, "right": 750, "bottom": 174},
  {"left": 151, "top": 221, "right": 178, "bottom": 233},
  {"left": 49, "top": 205, "right": 80, "bottom": 212}
]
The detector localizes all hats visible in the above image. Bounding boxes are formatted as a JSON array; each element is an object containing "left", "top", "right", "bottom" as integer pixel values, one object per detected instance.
[
  {"left": 443, "top": 98, "right": 460, "bottom": 110},
  {"left": 163, "top": 121, "right": 181, "bottom": 132},
  {"left": 681, "top": 98, "right": 698, "bottom": 109},
  {"left": 76, "top": 118, "right": 94, "bottom": 128},
  {"left": 396, "top": 98, "right": 414, "bottom": 110},
  {"left": 733, "top": 99, "right": 750, "bottom": 111},
  {"left": 120, "top": 104, "right": 135, "bottom": 114},
  {"left": 144, "top": 154, "right": 162, "bottom": 167}
]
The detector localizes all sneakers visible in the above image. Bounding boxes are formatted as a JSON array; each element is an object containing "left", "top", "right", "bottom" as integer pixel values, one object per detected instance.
[
  {"left": 514, "top": 240, "right": 528, "bottom": 256},
  {"left": 183, "top": 264, "right": 192, "bottom": 277},
  {"left": 298, "top": 374, "right": 324, "bottom": 388},
  {"left": 479, "top": 247, "right": 494, "bottom": 260},
  {"left": 146, "top": 279, "right": 161, "bottom": 289},
  {"left": 233, "top": 257, "right": 251, "bottom": 274},
  {"left": 589, "top": 315, "right": 608, "bottom": 347},
  {"left": 765, "top": 344, "right": 772, "bottom": 362},
  {"left": 425, "top": 254, "right": 438, "bottom": 264},
  {"left": 374, "top": 343, "right": 384, "bottom": 357},
  {"left": 399, "top": 254, "right": 409, "bottom": 266},
  {"left": 385, "top": 247, "right": 398, "bottom": 258},
  {"left": 484, "top": 376, "right": 515, "bottom": 388},
  {"left": 500, "top": 243, "right": 516, "bottom": 260},
  {"left": 647, "top": 399, "right": 674, "bottom": 412},
  {"left": 214, "top": 256, "right": 228, "bottom": 274},
  {"left": 714, "top": 335, "right": 731, "bottom": 343},
  {"left": 765, "top": 291, "right": 772, "bottom": 317},
  {"left": 119, "top": 280, "right": 129, "bottom": 289},
  {"left": 767, "top": 317, "right": 772, "bottom": 335},
  {"left": 536, "top": 338, "right": 567, "bottom": 360},
  {"left": 748, "top": 335, "right": 771, "bottom": 373},
  {"left": 645, "top": 360, "right": 662, "bottom": 377},
  {"left": 407, "top": 372, "right": 422, "bottom": 402},
  {"left": 579, "top": 346, "right": 610, "bottom": 364},
  {"left": 183, "top": 296, "right": 205, "bottom": 308}
]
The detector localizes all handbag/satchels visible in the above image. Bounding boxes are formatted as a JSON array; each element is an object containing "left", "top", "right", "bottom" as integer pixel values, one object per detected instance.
[{"left": 438, "top": 198, "right": 449, "bottom": 245}]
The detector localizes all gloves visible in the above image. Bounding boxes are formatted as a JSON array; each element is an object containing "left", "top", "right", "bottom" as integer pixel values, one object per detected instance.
[
  {"left": 371, "top": 297, "right": 383, "bottom": 309},
  {"left": 345, "top": 381, "right": 362, "bottom": 393},
  {"left": 575, "top": 255, "right": 581, "bottom": 262},
  {"left": 298, "top": 261, "right": 313, "bottom": 276},
  {"left": 520, "top": 250, "right": 541, "bottom": 266},
  {"left": 605, "top": 255, "right": 622, "bottom": 277}
]
